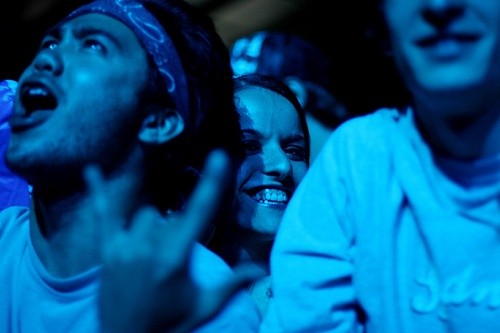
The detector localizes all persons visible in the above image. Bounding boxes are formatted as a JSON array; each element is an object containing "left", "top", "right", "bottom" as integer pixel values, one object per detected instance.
[
  {"left": 258, "top": 0, "right": 500, "bottom": 333},
  {"left": 1, "top": 0, "right": 260, "bottom": 333},
  {"left": 195, "top": 27, "right": 356, "bottom": 320}
]
